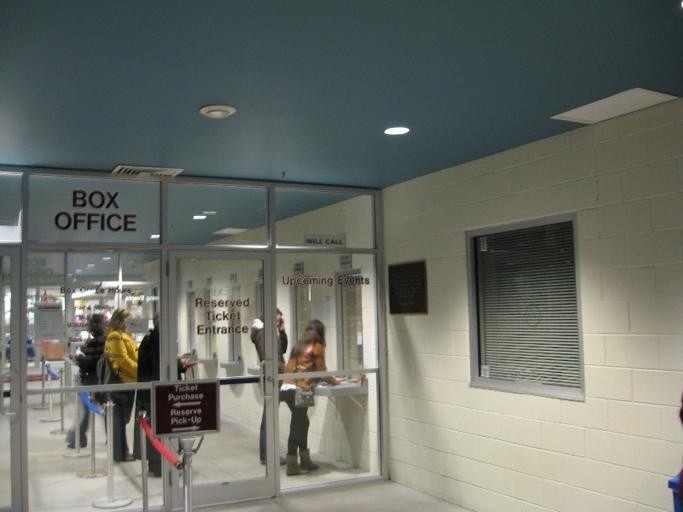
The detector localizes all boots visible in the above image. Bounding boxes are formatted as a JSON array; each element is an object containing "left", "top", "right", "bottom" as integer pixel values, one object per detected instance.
[
  {"left": 287, "top": 454, "right": 308, "bottom": 474},
  {"left": 300, "top": 450, "right": 318, "bottom": 469}
]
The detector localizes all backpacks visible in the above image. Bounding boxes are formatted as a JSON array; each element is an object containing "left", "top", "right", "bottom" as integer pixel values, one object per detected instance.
[{"left": 96, "top": 354, "right": 121, "bottom": 385}]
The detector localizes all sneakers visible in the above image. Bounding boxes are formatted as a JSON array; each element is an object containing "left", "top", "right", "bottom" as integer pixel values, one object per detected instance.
[
  {"left": 116, "top": 454, "right": 135, "bottom": 460},
  {"left": 265, "top": 457, "right": 285, "bottom": 465}
]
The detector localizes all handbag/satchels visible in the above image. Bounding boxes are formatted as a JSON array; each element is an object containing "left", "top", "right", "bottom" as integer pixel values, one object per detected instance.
[{"left": 296, "top": 386, "right": 315, "bottom": 408}]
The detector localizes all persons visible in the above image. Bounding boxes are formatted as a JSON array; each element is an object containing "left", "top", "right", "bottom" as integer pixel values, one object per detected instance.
[
  {"left": 134, "top": 312, "right": 194, "bottom": 477},
  {"left": 280, "top": 319, "right": 340, "bottom": 475},
  {"left": 251, "top": 308, "right": 287, "bottom": 466},
  {"left": 101, "top": 308, "right": 139, "bottom": 461},
  {"left": 64, "top": 311, "right": 110, "bottom": 447}
]
